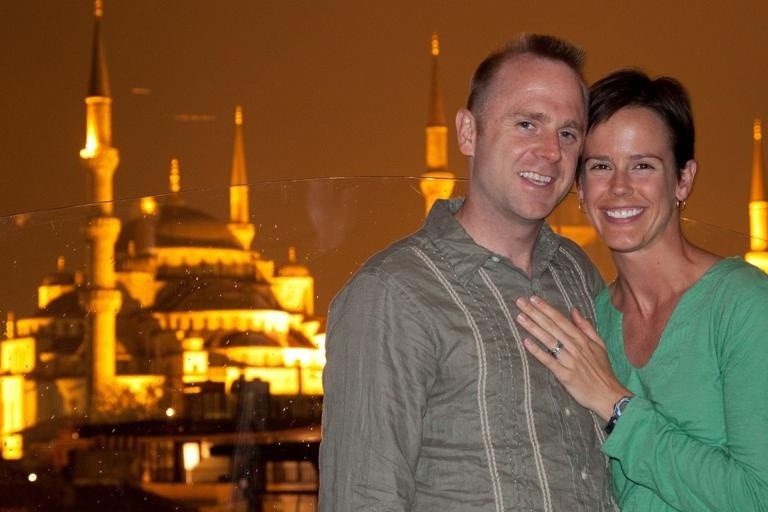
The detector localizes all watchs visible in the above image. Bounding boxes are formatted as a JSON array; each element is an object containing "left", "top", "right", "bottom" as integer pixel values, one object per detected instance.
[{"left": 604, "top": 395, "right": 634, "bottom": 435}]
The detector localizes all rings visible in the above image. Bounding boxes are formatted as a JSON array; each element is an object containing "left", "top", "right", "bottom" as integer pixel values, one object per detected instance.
[{"left": 552, "top": 340, "right": 562, "bottom": 355}]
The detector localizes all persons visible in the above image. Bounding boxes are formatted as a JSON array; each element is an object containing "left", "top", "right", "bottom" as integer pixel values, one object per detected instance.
[
  {"left": 316, "top": 34, "right": 622, "bottom": 511},
  {"left": 515, "top": 70, "right": 768, "bottom": 511}
]
